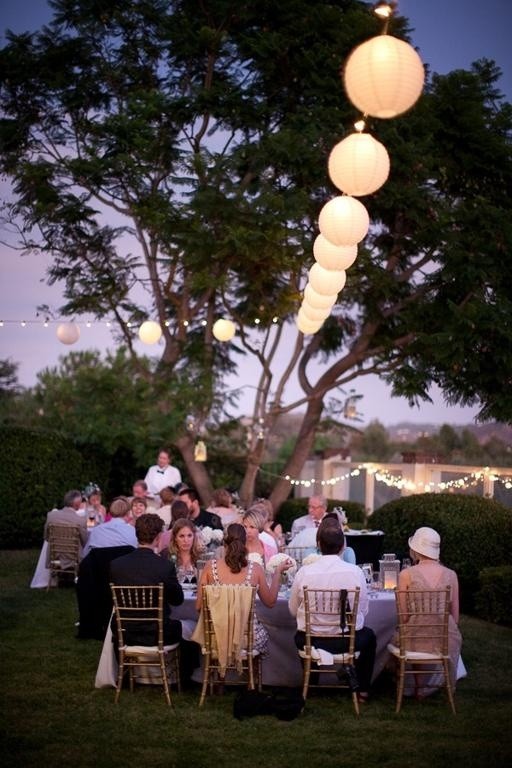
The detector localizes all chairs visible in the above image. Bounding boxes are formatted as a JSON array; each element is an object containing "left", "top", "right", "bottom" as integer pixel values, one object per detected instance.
[
  {"left": 196, "top": 583, "right": 264, "bottom": 709},
  {"left": 45, "top": 524, "right": 85, "bottom": 592},
  {"left": 386, "top": 585, "right": 456, "bottom": 716},
  {"left": 279, "top": 546, "right": 318, "bottom": 563},
  {"left": 298, "top": 585, "right": 360, "bottom": 716},
  {"left": 110, "top": 582, "right": 182, "bottom": 714}
]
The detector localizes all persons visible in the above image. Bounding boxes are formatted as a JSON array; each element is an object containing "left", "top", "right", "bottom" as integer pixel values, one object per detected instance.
[
  {"left": 111, "top": 513, "right": 184, "bottom": 677},
  {"left": 45, "top": 481, "right": 355, "bottom": 583},
  {"left": 196, "top": 523, "right": 294, "bottom": 695},
  {"left": 397, "top": 526, "right": 461, "bottom": 700},
  {"left": 145, "top": 452, "right": 181, "bottom": 501},
  {"left": 287, "top": 527, "right": 377, "bottom": 702}
]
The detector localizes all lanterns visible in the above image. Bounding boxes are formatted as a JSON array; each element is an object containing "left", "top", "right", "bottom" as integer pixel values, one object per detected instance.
[
  {"left": 57, "top": 324, "right": 80, "bottom": 343},
  {"left": 213, "top": 319, "right": 236, "bottom": 341},
  {"left": 329, "top": 133, "right": 390, "bottom": 196},
  {"left": 345, "top": 35, "right": 424, "bottom": 118},
  {"left": 139, "top": 321, "right": 162, "bottom": 343},
  {"left": 296, "top": 195, "right": 370, "bottom": 335}
]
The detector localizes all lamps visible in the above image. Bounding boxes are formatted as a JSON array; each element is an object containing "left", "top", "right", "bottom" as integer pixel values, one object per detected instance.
[
  {"left": 257, "top": 462, "right": 512, "bottom": 499},
  {"left": 51, "top": 316, "right": 243, "bottom": 347}
]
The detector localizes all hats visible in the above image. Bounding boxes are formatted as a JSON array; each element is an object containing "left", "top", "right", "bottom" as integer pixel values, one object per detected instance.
[{"left": 408, "top": 527, "right": 441, "bottom": 560}]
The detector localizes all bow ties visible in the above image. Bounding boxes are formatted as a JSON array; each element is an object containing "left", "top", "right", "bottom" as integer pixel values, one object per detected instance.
[{"left": 157, "top": 470, "right": 164, "bottom": 474}]
